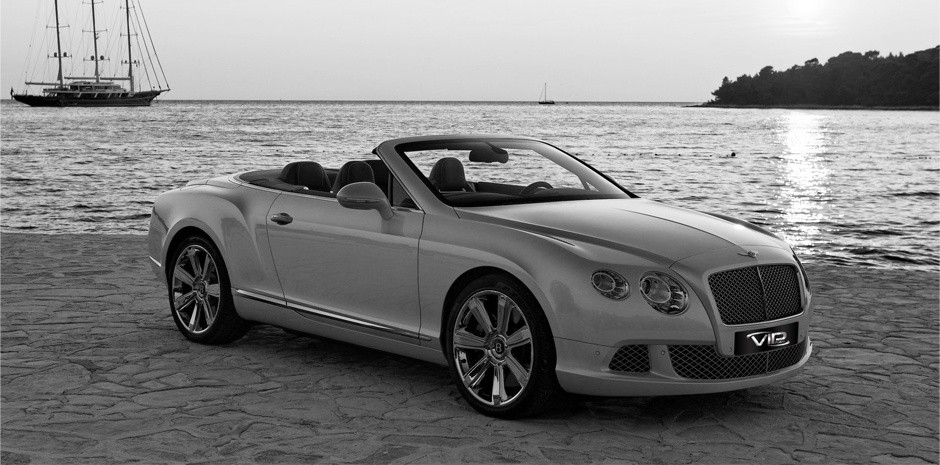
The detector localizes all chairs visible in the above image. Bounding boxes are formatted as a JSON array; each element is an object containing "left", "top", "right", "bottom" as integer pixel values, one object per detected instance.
[
  {"left": 328, "top": 160, "right": 374, "bottom": 195},
  {"left": 280, "top": 161, "right": 330, "bottom": 193},
  {"left": 430, "top": 157, "right": 474, "bottom": 194}
]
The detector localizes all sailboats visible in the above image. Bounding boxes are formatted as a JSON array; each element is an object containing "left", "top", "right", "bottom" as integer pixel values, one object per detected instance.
[
  {"left": 10, "top": 0, "right": 171, "bottom": 109},
  {"left": 538, "top": 80, "right": 556, "bottom": 105}
]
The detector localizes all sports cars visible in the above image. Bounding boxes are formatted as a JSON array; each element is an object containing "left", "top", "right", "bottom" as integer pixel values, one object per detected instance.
[{"left": 146, "top": 134, "right": 815, "bottom": 419}]
